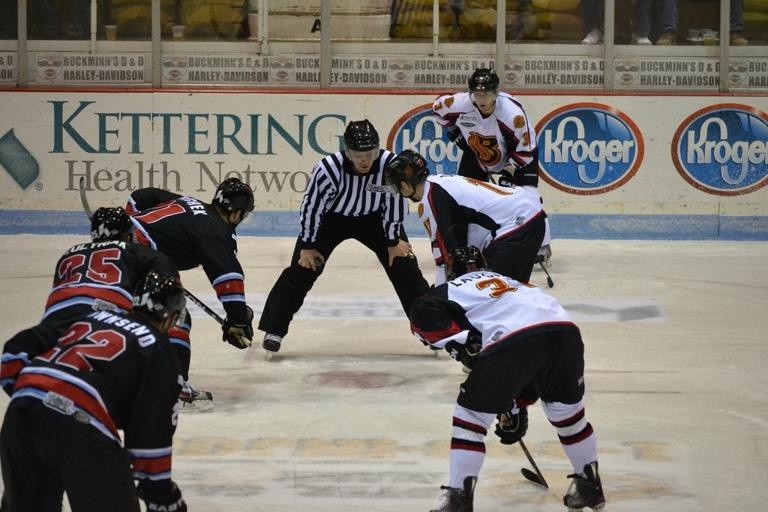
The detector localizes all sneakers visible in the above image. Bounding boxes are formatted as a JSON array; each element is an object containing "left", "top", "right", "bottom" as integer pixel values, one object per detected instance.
[
  {"left": 562, "top": 462, "right": 604, "bottom": 507},
  {"left": 535, "top": 246, "right": 552, "bottom": 264},
  {"left": 263, "top": 332, "right": 282, "bottom": 351},
  {"left": 178, "top": 382, "right": 211, "bottom": 402},
  {"left": 428, "top": 477, "right": 476, "bottom": 511}
]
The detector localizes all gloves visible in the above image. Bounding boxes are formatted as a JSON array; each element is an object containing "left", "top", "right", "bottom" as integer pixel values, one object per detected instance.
[
  {"left": 498, "top": 160, "right": 517, "bottom": 188},
  {"left": 496, "top": 407, "right": 528, "bottom": 444},
  {"left": 449, "top": 127, "right": 473, "bottom": 157},
  {"left": 222, "top": 306, "right": 253, "bottom": 349},
  {"left": 137, "top": 481, "right": 187, "bottom": 512}
]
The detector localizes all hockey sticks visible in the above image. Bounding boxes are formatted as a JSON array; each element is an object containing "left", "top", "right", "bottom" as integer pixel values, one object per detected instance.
[
  {"left": 471, "top": 152, "right": 553, "bottom": 288},
  {"left": 518, "top": 437, "right": 548, "bottom": 487},
  {"left": 80, "top": 178, "right": 252, "bottom": 346}
]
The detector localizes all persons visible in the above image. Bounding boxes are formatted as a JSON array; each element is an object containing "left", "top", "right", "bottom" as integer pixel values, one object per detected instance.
[
  {"left": 579, "top": 0, "right": 655, "bottom": 45},
  {"left": 410, "top": 244, "right": 606, "bottom": 512},
  {"left": 257, "top": 118, "right": 430, "bottom": 352},
  {"left": 0, "top": 269, "right": 188, "bottom": 512},
  {"left": 125, "top": 177, "right": 254, "bottom": 407},
  {"left": 41, "top": 208, "right": 180, "bottom": 326},
  {"left": 658, "top": 1, "right": 750, "bottom": 44},
  {"left": 385, "top": 150, "right": 548, "bottom": 371},
  {"left": 432, "top": 67, "right": 551, "bottom": 263}
]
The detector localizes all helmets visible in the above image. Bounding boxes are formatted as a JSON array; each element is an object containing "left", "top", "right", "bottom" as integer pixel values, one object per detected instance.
[
  {"left": 91, "top": 208, "right": 134, "bottom": 242},
  {"left": 132, "top": 271, "right": 187, "bottom": 329},
  {"left": 212, "top": 178, "right": 255, "bottom": 225},
  {"left": 385, "top": 149, "right": 429, "bottom": 200},
  {"left": 344, "top": 119, "right": 379, "bottom": 163},
  {"left": 445, "top": 245, "right": 488, "bottom": 279},
  {"left": 468, "top": 69, "right": 499, "bottom": 103}
]
